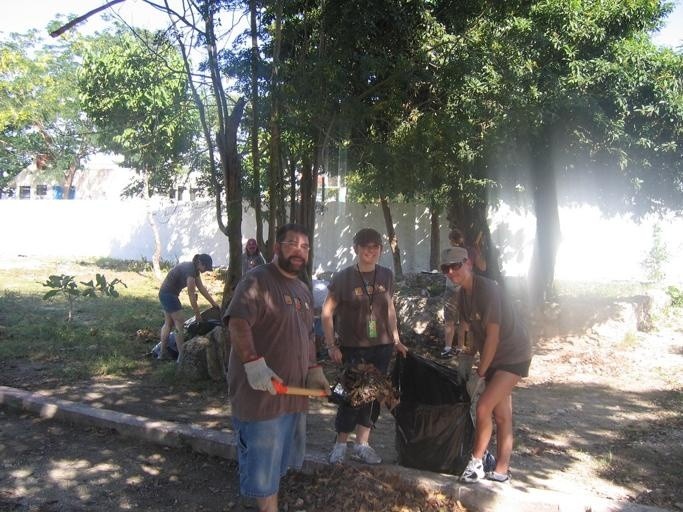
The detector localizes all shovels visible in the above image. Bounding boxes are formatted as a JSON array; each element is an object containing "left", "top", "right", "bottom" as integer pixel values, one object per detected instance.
[{"left": 272, "top": 380, "right": 376, "bottom": 409}]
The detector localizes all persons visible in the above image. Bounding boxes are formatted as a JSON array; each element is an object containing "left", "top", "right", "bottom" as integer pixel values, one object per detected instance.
[
  {"left": 157, "top": 253, "right": 217, "bottom": 364},
  {"left": 223, "top": 223, "right": 331, "bottom": 512},
  {"left": 439, "top": 228, "right": 487, "bottom": 359},
  {"left": 443, "top": 246, "right": 532, "bottom": 483},
  {"left": 242, "top": 237, "right": 264, "bottom": 276},
  {"left": 321, "top": 228, "right": 408, "bottom": 465}
]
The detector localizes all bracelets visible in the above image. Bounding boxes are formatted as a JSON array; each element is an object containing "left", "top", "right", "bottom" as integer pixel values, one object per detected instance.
[{"left": 325, "top": 340, "right": 337, "bottom": 349}]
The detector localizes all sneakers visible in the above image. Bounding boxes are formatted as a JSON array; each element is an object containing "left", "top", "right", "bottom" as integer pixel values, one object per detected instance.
[
  {"left": 330, "top": 443, "right": 348, "bottom": 464},
  {"left": 441, "top": 346, "right": 468, "bottom": 356},
  {"left": 460, "top": 457, "right": 486, "bottom": 483},
  {"left": 486, "top": 471, "right": 511, "bottom": 482},
  {"left": 157, "top": 350, "right": 183, "bottom": 363},
  {"left": 352, "top": 442, "right": 382, "bottom": 465}
]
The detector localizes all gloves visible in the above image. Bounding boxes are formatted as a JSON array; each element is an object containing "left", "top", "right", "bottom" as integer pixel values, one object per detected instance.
[
  {"left": 243, "top": 355, "right": 283, "bottom": 395},
  {"left": 307, "top": 365, "right": 332, "bottom": 399}
]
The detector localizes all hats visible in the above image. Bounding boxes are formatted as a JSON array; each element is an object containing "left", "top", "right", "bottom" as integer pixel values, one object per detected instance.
[
  {"left": 354, "top": 228, "right": 382, "bottom": 246},
  {"left": 440, "top": 247, "right": 468, "bottom": 265},
  {"left": 199, "top": 254, "right": 213, "bottom": 271}
]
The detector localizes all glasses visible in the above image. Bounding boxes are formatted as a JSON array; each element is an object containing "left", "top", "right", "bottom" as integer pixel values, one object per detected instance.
[
  {"left": 440, "top": 259, "right": 468, "bottom": 274},
  {"left": 278, "top": 240, "right": 310, "bottom": 252}
]
